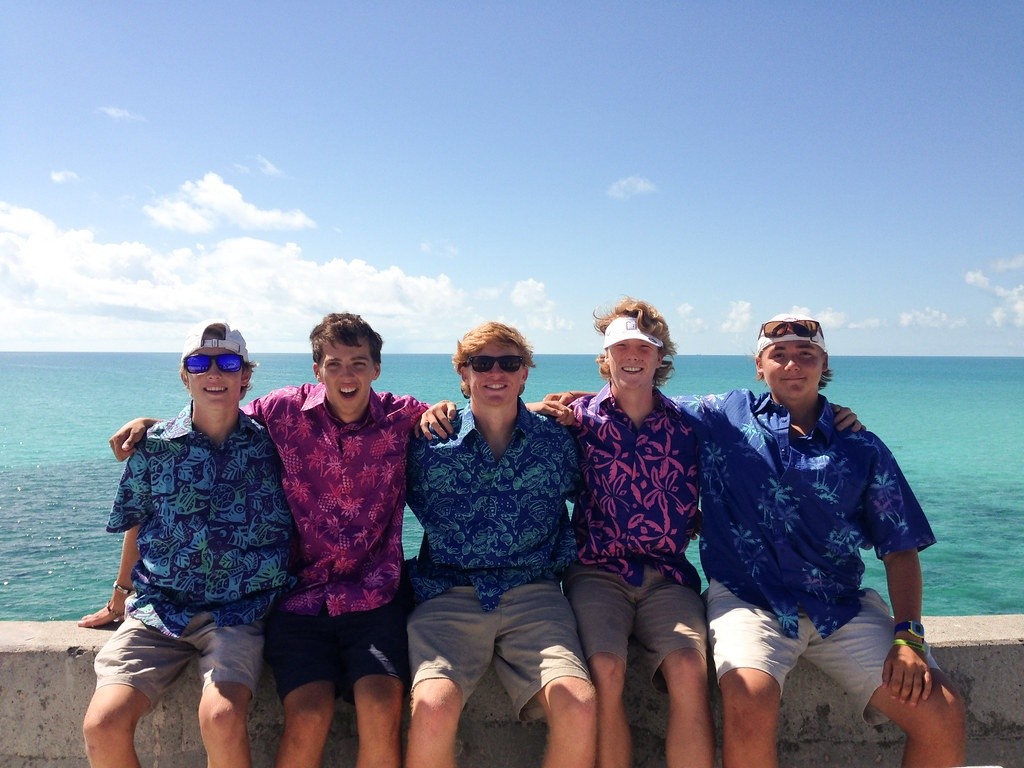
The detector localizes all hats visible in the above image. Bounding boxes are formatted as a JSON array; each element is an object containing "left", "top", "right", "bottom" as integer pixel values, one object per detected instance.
[
  {"left": 603, "top": 316, "right": 664, "bottom": 349},
  {"left": 756, "top": 313, "right": 826, "bottom": 356},
  {"left": 181, "top": 319, "right": 248, "bottom": 367}
]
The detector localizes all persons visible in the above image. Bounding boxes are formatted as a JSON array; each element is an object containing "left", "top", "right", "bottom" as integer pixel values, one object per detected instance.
[
  {"left": 108, "top": 312, "right": 576, "bottom": 768},
  {"left": 403, "top": 321, "right": 598, "bottom": 768},
  {"left": 77, "top": 320, "right": 294, "bottom": 768},
  {"left": 544, "top": 314, "right": 966, "bottom": 768},
  {"left": 414, "top": 297, "right": 868, "bottom": 768}
]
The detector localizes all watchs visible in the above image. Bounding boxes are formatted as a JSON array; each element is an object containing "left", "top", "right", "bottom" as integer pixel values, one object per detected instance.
[{"left": 895, "top": 620, "right": 924, "bottom": 643}]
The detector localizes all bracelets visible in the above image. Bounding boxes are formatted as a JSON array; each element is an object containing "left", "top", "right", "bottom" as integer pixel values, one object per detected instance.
[
  {"left": 107, "top": 601, "right": 122, "bottom": 616},
  {"left": 569, "top": 392, "right": 576, "bottom": 399},
  {"left": 113, "top": 582, "right": 130, "bottom": 594},
  {"left": 892, "top": 639, "right": 926, "bottom": 654}
]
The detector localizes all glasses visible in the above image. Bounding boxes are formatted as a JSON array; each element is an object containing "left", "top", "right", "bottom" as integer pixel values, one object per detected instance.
[
  {"left": 467, "top": 355, "right": 525, "bottom": 372},
  {"left": 184, "top": 353, "right": 244, "bottom": 374},
  {"left": 758, "top": 319, "right": 825, "bottom": 340}
]
